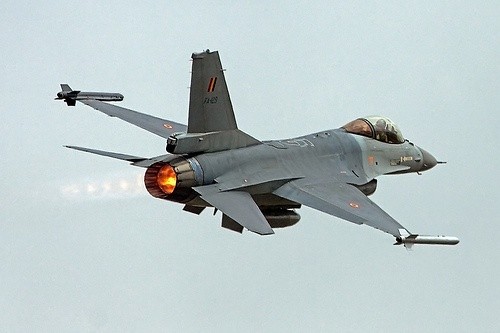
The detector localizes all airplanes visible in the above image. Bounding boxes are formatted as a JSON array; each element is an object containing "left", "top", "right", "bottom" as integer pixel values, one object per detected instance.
[{"left": 54, "top": 49, "right": 460, "bottom": 250}]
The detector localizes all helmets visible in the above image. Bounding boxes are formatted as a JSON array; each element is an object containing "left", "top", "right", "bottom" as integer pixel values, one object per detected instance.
[{"left": 375, "top": 119, "right": 386, "bottom": 127}]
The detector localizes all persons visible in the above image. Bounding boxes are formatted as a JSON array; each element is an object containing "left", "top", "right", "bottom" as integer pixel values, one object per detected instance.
[{"left": 372, "top": 119, "right": 386, "bottom": 137}]
